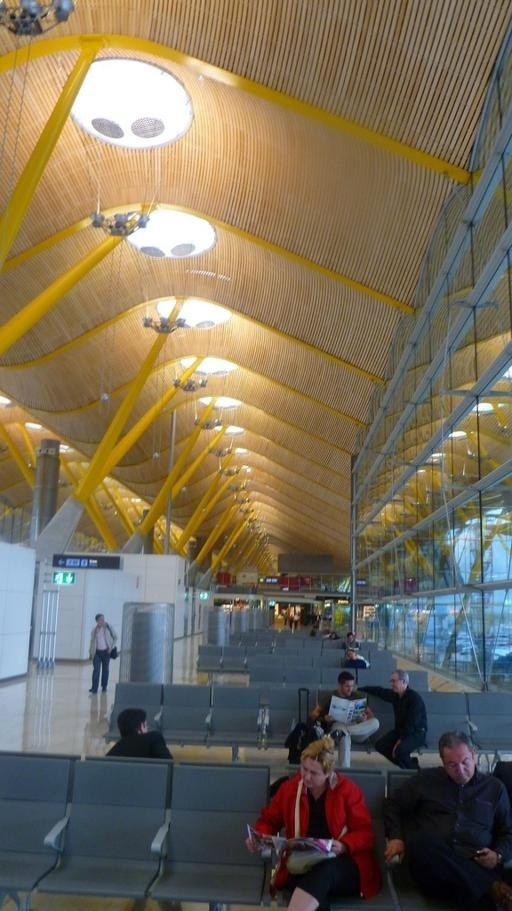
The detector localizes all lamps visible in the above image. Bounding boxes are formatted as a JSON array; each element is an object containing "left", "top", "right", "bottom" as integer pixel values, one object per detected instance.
[
  {"left": 183, "top": 354, "right": 252, "bottom": 475},
  {"left": 156, "top": 298, "right": 231, "bottom": 328},
  {"left": 67, "top": 58, "right": 192, "bottom": 149},
  {"left": 416, "top": 363, "right": 511, "bottom": 474},
  {"left": 124, "top": 210, "right": 216, "bottom": 258}
]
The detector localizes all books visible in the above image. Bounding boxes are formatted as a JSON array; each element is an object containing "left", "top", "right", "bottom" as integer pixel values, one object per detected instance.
[
  {"left": 246, "top": 824, "right": 336, "bottom": 878},
  {"left": 327, "top": 693, "right": 370, "bottom": 728}
]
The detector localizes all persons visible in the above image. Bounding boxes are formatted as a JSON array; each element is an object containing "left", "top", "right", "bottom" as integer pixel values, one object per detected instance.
[
  {"left": 280, "top": 609, "right": 341, "bottom": 640},
  {"left": 355, "top": 669, "right": 428, "bottom": 775},
  {"left": 85, "top": 613, "right": 120, "bottom": 697},
  {"left": 103, "top": 707, "right": 173, "bottom": 762},
  {"left": 379, "top": 729, "right": 511, "bottom": 910},
  {"left": 342, "top": 651, "right": 367, "bottom": 670},
  {"left": 309, "top": 670, "right": 380, "bottom": 772},
  {"left": 243, "top": 734, "right": 384, "bottom": 910},
  {"left": 341, "top": 632, "right": 361, "bottom": 652}
]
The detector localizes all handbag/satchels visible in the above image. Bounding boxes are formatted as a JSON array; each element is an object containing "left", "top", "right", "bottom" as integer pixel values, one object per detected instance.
[
  {"left": 286, "top": 849, "right": 335, "bottom": 875},
  {"left": 110, "top": 646, "right": 117, "bottom": 659}
]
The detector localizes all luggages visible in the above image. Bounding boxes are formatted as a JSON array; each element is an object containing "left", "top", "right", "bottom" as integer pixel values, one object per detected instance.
[{"left": 287, "top": 687, "right": 318, "bottom": 761}]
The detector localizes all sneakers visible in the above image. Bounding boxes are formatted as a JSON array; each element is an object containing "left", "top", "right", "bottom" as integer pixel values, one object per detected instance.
[
  {"left": 89, "top": 688, "right": 97, "bottom": 693},
  {"left": 102, "top": 687, "right": 106, "bottom": 691}
]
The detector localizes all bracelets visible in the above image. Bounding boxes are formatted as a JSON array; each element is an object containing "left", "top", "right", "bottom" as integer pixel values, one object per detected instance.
[{"left": 495, "top": 850, "right": 501, "bottom": 868}]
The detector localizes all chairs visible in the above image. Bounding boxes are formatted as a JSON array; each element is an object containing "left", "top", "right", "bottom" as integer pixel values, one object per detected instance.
[
  {"left": 1, "top": 751, "right": 427, "bottom": 911},
  {"left": 196, "top": 629, "right": 429, "bottom": 689},
  {"left": 103, "top": 683, "right": 512, "bottom": 772}
]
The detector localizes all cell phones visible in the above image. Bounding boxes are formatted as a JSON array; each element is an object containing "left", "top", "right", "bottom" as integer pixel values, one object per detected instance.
[{"left": 470, "top": 849, "right": 484, "bottom": 859}]
